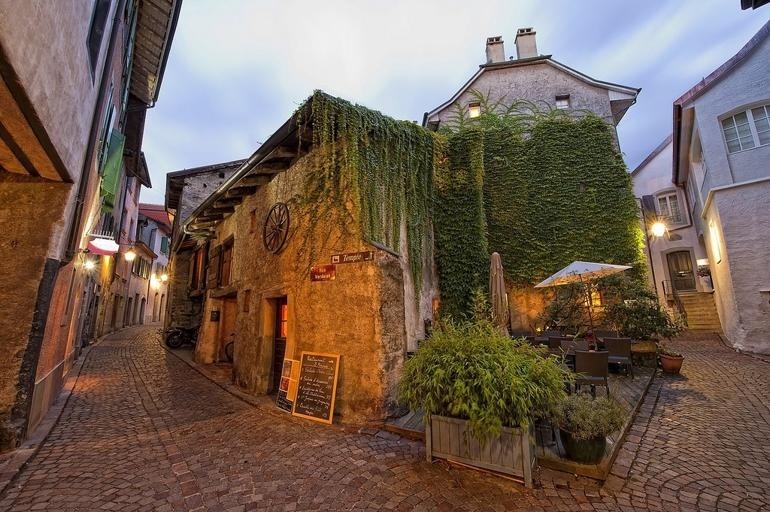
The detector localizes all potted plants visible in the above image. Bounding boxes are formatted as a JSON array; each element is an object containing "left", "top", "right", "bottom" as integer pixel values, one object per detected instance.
[
  {"left": 697, "top": 264, "right": 714, "bottom": 293},
  {"left": 391, "top": 286, "right": 564, "bottom": 488},
  {"left": 551, "top": 379, "right": 629, "bottom": 466}
]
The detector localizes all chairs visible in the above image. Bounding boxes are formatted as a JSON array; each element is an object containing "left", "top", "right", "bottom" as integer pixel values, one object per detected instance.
[{"left": 525, "top": 337, "right": 634, "bottom": 400}]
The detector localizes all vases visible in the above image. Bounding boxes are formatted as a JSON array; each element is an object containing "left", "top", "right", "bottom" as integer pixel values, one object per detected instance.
[{"left": 659, "top": 352, "right": 684, "bottom": 375}]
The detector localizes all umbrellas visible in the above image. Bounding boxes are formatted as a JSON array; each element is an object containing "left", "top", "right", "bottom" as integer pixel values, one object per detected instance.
[
  {"left": 533, "top": 260, "right": 637, "bottom": 349},
  {"left": 487, "top": 249, "right": 512, "bottom": 329}
]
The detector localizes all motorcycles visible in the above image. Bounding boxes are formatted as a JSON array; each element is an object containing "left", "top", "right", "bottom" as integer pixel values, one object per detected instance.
[{"left": 165, "top": 323, "right": 200, "bottom": 347}]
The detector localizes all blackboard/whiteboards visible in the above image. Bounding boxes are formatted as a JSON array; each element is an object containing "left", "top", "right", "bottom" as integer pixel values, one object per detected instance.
[{"left": 291, "top": 351, "right": 340, "bottom": 424}]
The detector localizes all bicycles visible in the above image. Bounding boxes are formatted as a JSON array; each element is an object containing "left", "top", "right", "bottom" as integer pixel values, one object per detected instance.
[{"left": 225, "top": 333, "right": 234, "bottom": 361}]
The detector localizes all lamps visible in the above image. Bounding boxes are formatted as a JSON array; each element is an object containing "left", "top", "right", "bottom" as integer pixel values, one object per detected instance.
[{"left": 124, "top": 244, "right": 137, "bottom": 262}]
